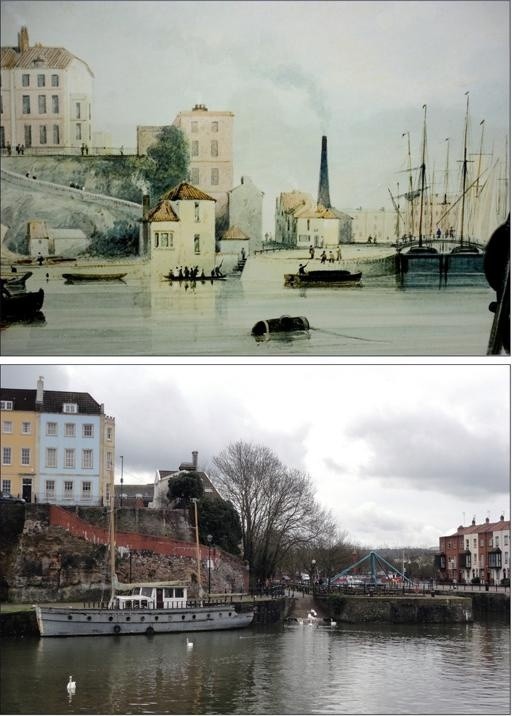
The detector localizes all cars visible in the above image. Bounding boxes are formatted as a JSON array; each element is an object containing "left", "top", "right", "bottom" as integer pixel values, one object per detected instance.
[
  {"left": 301, "top": 574, "right": 310, "bottom": 581},
  {"left": 0, "top": 491, "right": 26, "bottom": 505},
  {"left": 282, "top": 575, "right": 291, "bottom": 582}
]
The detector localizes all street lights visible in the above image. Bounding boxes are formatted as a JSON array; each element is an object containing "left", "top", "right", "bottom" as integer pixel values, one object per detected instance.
[
  {"left": 310, "top": 559, "right": 318, "bottom": 593},
  {"left": 119, "top": 455, "right": 124, "bottom": 507},
  {"left": 207, "top": 534, "right": 213, "bottom": 594}
]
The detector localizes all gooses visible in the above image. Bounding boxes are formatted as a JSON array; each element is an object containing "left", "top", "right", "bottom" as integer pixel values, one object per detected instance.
[
  {"left": 186, "top": 637, "right": 193, "bottom": 646},
  {"left": 67, "top": 676, "right": 76, "bottom": 689},
  {"left": 330, "top": 618, "right": 336, "bottom": 625},
  {"left": 296, "top": 608, "right": 317, "bottom": 626}
]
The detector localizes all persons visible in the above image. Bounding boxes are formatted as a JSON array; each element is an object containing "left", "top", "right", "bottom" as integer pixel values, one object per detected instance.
[
  {"left": 242, "top": 248, "right": 246, "bottom": 259},
  {"left": 297, "top": 261, "right": 310, "bottom": 273},
  {"left": 366, "top": 226, "right": 456, "bottom": 244},
  {"left": 167, "top": 263, "right": 223, "bottom": 279},
  {"left": 24, "top": 172, "right": 37, "bottom": 179},
  {"left": 81, "top": 143, "right": 88, "bottom": 156},
  {"left": 67, "top": 182, "right": 86, "bottom": 190},
  {"left": 5, "top": 139, "right": 26, "bottom": 155},
  {"left": 308, "top": 244, "right": 344, "bottom": 262}
]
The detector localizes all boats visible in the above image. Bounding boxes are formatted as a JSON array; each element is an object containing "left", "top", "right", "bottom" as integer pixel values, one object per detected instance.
[{"left": 35, "top": 584, "right": 256, "bottom": 639}]
